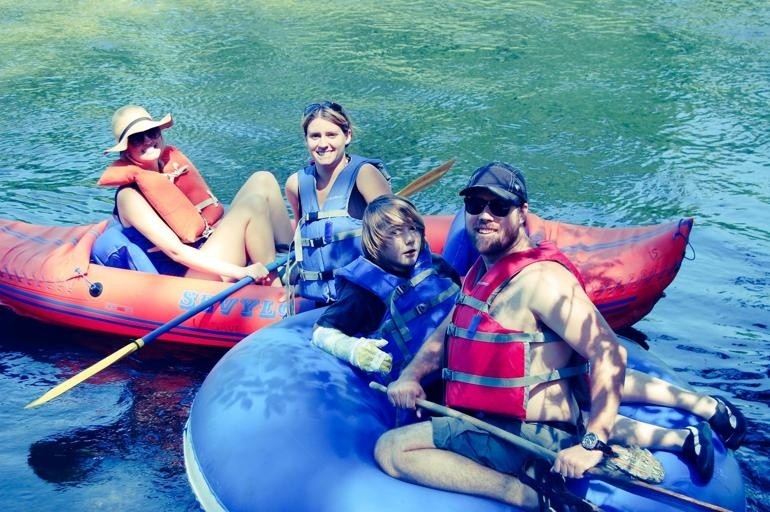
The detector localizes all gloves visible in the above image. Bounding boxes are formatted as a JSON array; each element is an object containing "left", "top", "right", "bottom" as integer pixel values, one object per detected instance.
[{"left": 311, "top": 325, "right": 392, "bottom": 374}]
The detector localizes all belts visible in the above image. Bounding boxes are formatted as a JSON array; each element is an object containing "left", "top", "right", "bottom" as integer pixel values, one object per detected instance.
[{"left": 525, "top": 411, "right": 584, "bottom": 435}]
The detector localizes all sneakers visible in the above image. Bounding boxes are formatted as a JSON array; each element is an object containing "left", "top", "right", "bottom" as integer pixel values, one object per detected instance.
[
  {"left": 520, "top": 456, "right": 599, "bottom": 512},
  {"left": 680, "top": 421, "right": 714, "bottom": 487},
  {"left": 708, "top": 394, "right": 746, "bottom": 450}
]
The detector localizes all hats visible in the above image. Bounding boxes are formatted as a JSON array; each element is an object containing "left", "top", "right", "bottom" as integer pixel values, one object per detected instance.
[
  {"left": 103, "top": 104, "right": 174, "bottom": 156},
  {"left": 460, "top": 162, "right": 528, "bottom": 205}
]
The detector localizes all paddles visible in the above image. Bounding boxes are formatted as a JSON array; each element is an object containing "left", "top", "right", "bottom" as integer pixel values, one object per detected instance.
[{"left": 23, "top": 159, "right": 456, "bottom": 410}]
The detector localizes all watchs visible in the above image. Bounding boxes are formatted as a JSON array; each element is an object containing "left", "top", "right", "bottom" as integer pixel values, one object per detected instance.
[{"left": 581, "top": 432, "right": 611, "bottom": 454}]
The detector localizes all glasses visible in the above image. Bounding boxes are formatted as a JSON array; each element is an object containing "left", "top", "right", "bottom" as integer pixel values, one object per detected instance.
[
  {"left": 128, "top": 127, "right": 161, "bottom": 147},
  {"left": 304, "top": 103, "right": 342, "bottom": 117},
  {"left": 464, "top": 197, "right": 511, "bottom": 217}
]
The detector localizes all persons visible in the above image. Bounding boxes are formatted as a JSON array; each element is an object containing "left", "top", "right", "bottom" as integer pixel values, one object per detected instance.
[
  {"left": 284, "top": 102, "right": 392, "bottom": 305},
  {"left": 315, "top": 193, "right": 747, "bottom": 476},
  {"left": 99, "top": 103, "right": 294, "bottom": 288},
  {"left": 373, "top": 161, "right": 665, "bottom": 511}
]
